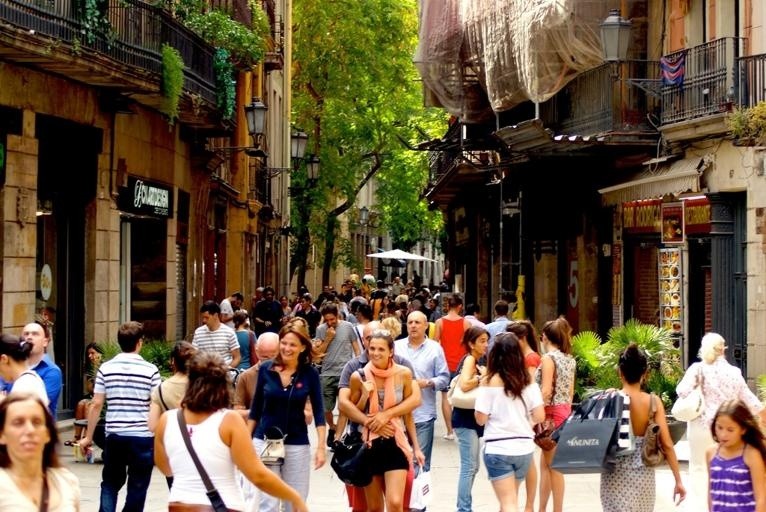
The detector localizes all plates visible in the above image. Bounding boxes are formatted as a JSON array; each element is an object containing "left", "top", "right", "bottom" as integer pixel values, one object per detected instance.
[
  {"left": 670, "top": 266, "right": 680, "bottom": 278},
  {"left": 663, "top": 293, "right": 669, "bottom": 304},
  {"left": 662, "top": 267, "right": 670, "bottom": 278},
  {"left": 672, "top": 294, "right": 680, "bottom": 301},
  {"left": 664, "top": 307, "right": 673, "bottom": 319},
  {"left": 672, "top": 322, "right": 682, "bottom": 332}
]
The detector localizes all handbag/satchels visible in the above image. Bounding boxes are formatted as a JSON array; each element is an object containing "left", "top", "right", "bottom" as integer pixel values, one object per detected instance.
[
  {"left": 642, "top": 421, "right": 667, "bottom": 465},
  {"left": 551, "top": 393, "right": 636, "bottom": 474},
  {"left": 331, "top": 435, "right": 373, "bottom": 487},
  {"left": 409, "top": 471, "right": 431, "bottom": 509},
  {"left": 534, "top": 422, "right": 556, "bottom": 451},
  {"left": 671, "top": 386, "right": 704, "bottom": 422},
  {"left": 447, "top": 373, "right": 481, "bottom": 409},
  {"left": 251, "top": 437, "right": 285, "bottom": 465}
]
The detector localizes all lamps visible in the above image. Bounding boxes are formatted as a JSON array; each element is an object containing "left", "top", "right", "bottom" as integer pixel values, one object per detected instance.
[
  {"left": 598, "top": 8, "right": 661, "bottom": 97},
  {"left": 289, "top": 154, "right": 321, "bottom": 188},
  {"left": 215, "top": 96, "right": 268, "bottom": 160},
  {"left": 265, "top": 127, "right": 308, "bottom": 171},
  {"left": 352, "top": 206, "right": 369, "bottom": 225}
]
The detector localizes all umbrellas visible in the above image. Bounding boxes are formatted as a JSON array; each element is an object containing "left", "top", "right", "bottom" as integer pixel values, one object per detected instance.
[{"left": 364, "top": 248, "right": 440, "bottom": 263}]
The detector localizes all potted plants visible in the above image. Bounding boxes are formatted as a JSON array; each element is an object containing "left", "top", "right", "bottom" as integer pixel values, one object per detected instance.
[
  {"left": 74, "top": 336, "right": 177, "bottom": 463},
  {"left": 569, "top": 317, "right": 688, "bottom": 447}
]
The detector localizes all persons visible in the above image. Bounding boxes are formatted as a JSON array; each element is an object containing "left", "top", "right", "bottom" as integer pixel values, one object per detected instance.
[
  {"left": 591, "top": 342, "right": 687, "bottom": 512},
  {"left": 705, "top": 398, "right": 765, "bottom": 512},
  {"left": 64, "top": 272, "right": 576, "bottom": 512},
  {"left": 1, "top": 323, "right": 80, "bottom": 512},
  {"left": 676, "top": 332, "right": 766, "bottom": 512}
]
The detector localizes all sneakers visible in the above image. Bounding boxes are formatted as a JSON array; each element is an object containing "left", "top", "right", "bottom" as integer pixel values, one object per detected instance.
[{"left": 443, "top": 434, "right": 454, "bottom": 440}]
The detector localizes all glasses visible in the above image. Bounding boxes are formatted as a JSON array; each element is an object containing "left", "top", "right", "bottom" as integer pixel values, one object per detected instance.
[{"left": 372, "top": 329, "right": 392, "bottom": 337}]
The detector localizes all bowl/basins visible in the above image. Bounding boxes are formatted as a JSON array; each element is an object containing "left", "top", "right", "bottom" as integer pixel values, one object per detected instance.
[
  {"left": 138, "top": 282, "right": 166, "bottom": 292},
  {"left": 131, "top": 300, "right": 158, "bottom": 309}
]
